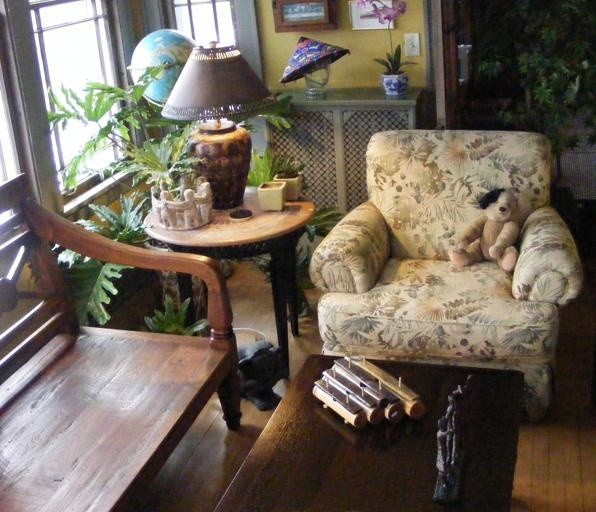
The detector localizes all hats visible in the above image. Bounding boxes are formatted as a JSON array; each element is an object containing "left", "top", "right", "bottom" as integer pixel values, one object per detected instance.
[{"left": 279, "top": 36, "right": 349, "bottom": 86}]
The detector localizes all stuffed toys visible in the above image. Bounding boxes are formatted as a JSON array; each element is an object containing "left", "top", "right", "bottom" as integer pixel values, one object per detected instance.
[{"left": 448, "top": 190, "right": 519, "bottom": 273}]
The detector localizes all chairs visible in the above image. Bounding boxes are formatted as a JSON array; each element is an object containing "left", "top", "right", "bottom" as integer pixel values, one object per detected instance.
[
  {"left": 0, "top": 171, "right": 241, "bottom": 511},
  {"left": 310, "top": 130, "right": 584, "bottom": 421}
]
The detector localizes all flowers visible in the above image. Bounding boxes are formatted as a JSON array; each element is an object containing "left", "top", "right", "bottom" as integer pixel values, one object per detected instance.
[{"left": 355, "top": 0, "right": 421, "bottom": 74}]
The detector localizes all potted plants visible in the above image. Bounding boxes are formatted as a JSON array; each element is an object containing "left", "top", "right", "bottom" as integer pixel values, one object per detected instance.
[{"left": 274, "top": 154, "right": 309, "bottom": 200}]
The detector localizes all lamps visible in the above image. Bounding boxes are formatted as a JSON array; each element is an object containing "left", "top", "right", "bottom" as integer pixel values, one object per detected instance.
[{"left": 162, "top": 42, "right": 280, "bottom": 209}]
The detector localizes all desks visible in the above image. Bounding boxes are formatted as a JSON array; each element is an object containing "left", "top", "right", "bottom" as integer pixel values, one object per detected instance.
[{"left": 210, "top": 354, "right": 525, "bottom": 510}]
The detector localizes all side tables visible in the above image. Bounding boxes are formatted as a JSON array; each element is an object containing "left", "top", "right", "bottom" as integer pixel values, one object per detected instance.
[{"left": 142, "top": 193, "right": 315, "bottom": 379}]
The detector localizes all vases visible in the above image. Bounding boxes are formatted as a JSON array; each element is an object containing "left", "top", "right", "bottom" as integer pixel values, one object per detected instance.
[
  {"left": 258, "top": 182, "right": 287, "bottom": 210},
  {"left": 380, "top": 70, "right": 409, "bottom": 97}
]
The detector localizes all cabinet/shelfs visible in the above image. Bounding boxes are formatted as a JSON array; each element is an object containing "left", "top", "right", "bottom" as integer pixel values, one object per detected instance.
[{"left": 271, "top": 0, "right": 339, "bottom": 33}]
